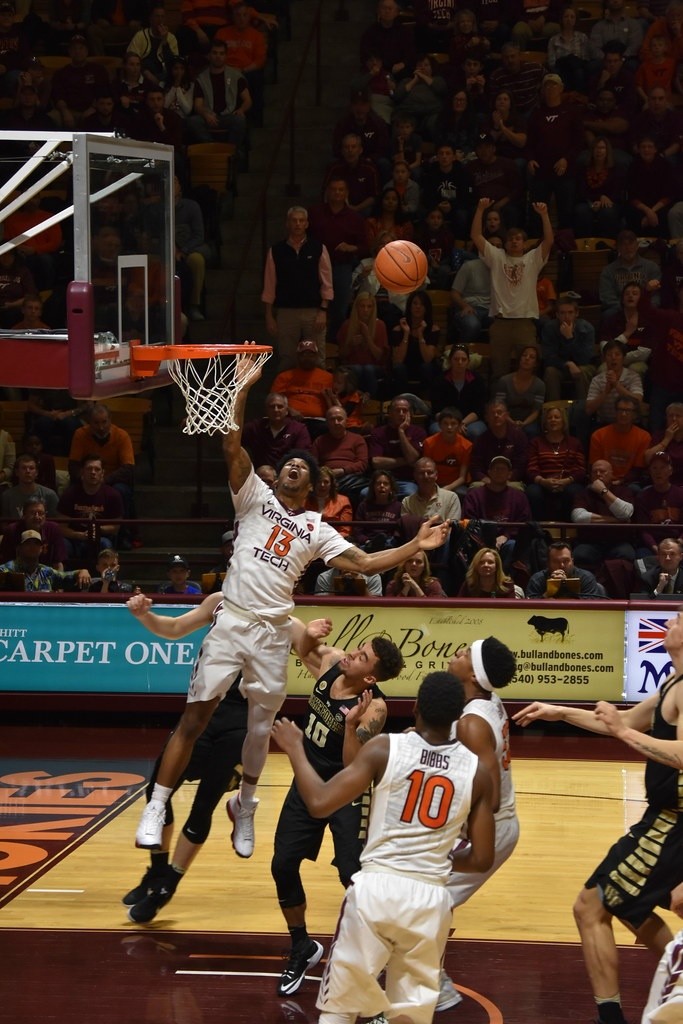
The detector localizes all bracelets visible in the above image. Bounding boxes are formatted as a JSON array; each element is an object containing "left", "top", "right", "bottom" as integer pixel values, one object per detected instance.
[
  {"left": 319, "top": 306, "right": 328, "bottom": 311},
  {"left": 568, "top": 477, "right": 572, "bottom": 484},
  {"left": 538, "top": 477, "right": 543, "bottom": 484},
  {"left": 661, "top": 151, "right": 666, "bottom": 157}
]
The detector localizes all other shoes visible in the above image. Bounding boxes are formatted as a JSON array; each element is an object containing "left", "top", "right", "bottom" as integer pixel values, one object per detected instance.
[
  {"left": 190, "top": 308, "right": 204, "bottom": 321},
  {"left": 434, "top": 976, "right": 462, "bottom": 1012},
  {"left": 365, "top": 1017, "right": 389, "bottom": 1024},
  {"left": 131, "top": 537, "right": 144, "bottom": 549}
]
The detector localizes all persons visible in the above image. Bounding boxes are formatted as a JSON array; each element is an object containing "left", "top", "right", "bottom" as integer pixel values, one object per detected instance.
[
  {"left": 271, "top": 617, "right": 519, "bottom": 1024},
  {"left": 0, "top": 0, "right": 683, "bottom": 600},
  {"left": 511, "top": 608, "right": 683, "bottom": 1024},
  {"left": 121, "top": 338, "right": 452, "bottom": 924}
]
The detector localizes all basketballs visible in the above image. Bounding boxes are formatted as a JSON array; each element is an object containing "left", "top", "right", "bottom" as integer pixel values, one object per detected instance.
[{"left": 374, "top": 240, "right": 427, "bottom": 295}]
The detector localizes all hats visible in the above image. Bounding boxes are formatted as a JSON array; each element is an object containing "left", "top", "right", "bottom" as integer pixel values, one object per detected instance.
[
  {"left": 222, "top": 530, "right": 234, "bottom": 543},
  {"left": 71, "top": 32, "right": 88, "bottom": 45},
  {"left": 20, "top": 530, "right": 41, "bottom": 543},
  {"left": 0, "top": 2, "right": 15, "bottom": 16},
  {"left": 296, "top": 340, "right": 318, "bottom": 354},
  {"left": 166, "top": 556, "right": 189, "bottom": 571},
  {"left": 25, "top": 56, "right": 44, "bottom": 67},
  {"left": 350, "top": 89, "right": 370, "bottom": 101},
  {"left": 488, "top": 450, "right": 512, "bottom": 466},
  {"left": 541, "top": 73, "right": 562, "bottom": 87},
  {"left": 474, "top": 131, "right": 494, "bottom": 146}
]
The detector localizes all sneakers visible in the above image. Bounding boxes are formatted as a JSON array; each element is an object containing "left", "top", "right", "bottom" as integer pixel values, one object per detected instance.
[
  {"left": 277, "top": 940, "right": 324, "bottom": 997},
  {"left": 122, "top": 870, "right": 165, "bottom": 906},
  {"left": 226, "top": 792, "right": 260, "bottom": 859},
  {"left": 128, "top": 885, "right": 174, "bottom": 924},
  {"left": 135, "top": 803, "right": 166, "bottom": 849}
]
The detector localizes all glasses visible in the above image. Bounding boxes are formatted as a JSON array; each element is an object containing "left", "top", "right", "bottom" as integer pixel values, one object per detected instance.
[
  {"left": 454, "top": 344, "right": 468, "bottom": 351},
  {"left": 617, "top": 407, "right": 636, "bottom": 413},
  {"left": 26, "top": 510, "right": 48, "bottom": 518}
]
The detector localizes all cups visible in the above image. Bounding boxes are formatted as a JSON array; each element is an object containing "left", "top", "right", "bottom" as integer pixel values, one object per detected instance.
[{"left": 88, "top": 523, "right": 100, "bottom": 542}]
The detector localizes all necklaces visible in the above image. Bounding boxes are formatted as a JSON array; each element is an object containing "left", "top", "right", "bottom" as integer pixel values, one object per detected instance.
[{"left": 548, "top": 436, "right": 562, "bottom": 456}]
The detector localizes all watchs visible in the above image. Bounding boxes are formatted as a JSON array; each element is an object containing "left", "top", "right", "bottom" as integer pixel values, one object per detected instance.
[{"left": 599, "top": 488, "right": 608, "bottom": 495}]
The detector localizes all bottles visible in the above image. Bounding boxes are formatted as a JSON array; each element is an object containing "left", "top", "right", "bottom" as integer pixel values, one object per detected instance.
[{"left": 106, "top": 570, "right": 115, "bottom": 583}]
